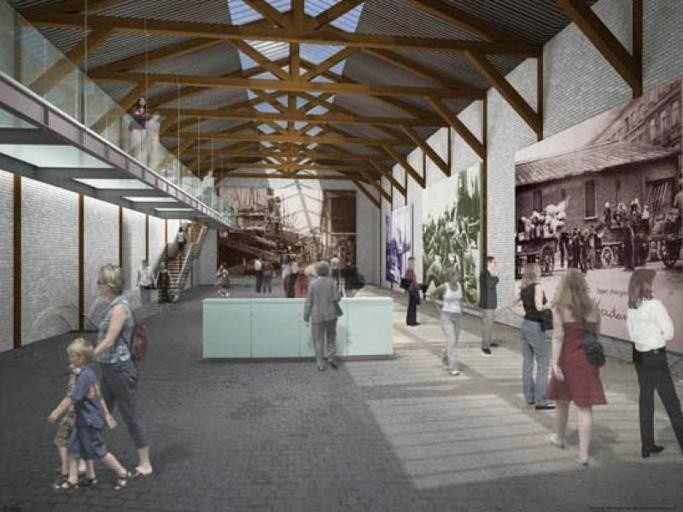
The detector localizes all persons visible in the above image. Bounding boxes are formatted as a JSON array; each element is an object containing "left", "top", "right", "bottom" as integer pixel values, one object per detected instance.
[
  {"left": 215, "top": 262, "right": 230, "bottom": 297},
  {"left": 144, "top": 111, "right": 160, "bottom": 143},
  {"left": 47, "top": 260, "right": 172, "bottom": 493},
  {"left": 254, "top": 245, "right": 344, "bottom": 373},
  {"left": 625, "top": 269, "right": 682, "bottom": 458},
  {"left": 509, "top": 263, "right": 555, "bottom": 410},
  {"left": 187, "top": 223, "right": 194, "bottom": 242},
  {"left": 176, "top": 227, "right": 187, "bottom": 251},
  {"left": 132, "top": 96, "right": 150, "bottom": 128},
  {"left": 421, "top": 206, "right": 479, "bottom": 305},
  {"left": 403, "top": 257, "right": 424, "bottom": 326},
  {"left": 479, "top": 257, "right": 501, "bottom": 355},
  {"left": 203, "top": 169, "right": 214, "bottom": 189},
  {"left": 515, "top": 177, "right": 682, "bottom": 276},
  {"left": 547, "top": 268, "right": 608, "bottom": 466},
  {"left": 429, "top": 267, "right": 466, "bottom": 376}
]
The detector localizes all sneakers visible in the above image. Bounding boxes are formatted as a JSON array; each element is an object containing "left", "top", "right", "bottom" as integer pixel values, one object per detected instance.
[
  {"left": 491, "top": 342, "right": 499, "bottom": 346},
  {"left": 483, "top": 347, "right": 490, "bottom": 354}
]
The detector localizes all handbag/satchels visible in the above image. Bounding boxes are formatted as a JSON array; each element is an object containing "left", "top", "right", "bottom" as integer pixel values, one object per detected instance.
[
  {"left": 334, "top": 301, "right": 343, "bottom": 317},
  {"left": 400, "top": 278, "right": 411, "bottom": 290},
  {"left": 580, "top": 315, "right": 606, "bottom": 367}
]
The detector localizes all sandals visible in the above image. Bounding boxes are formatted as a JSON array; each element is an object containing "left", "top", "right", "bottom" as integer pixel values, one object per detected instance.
[
  {"left": 113, "top": 471, "right": 131, "bottom": 492},
  {"left": 51, "top": 469, "right": 98, "bottom": 490}
]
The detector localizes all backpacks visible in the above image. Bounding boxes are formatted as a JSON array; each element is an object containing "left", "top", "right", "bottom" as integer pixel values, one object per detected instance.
[{"left": 112, "top": 302, "right": 148, "bottom": 360}]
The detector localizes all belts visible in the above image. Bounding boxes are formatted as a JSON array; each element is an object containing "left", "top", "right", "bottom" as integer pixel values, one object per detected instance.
[{"left": 640, "top": 348, "right": 665, "bottom": 355}]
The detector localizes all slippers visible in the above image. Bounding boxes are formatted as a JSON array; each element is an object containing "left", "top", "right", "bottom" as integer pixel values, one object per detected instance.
[{"left": 132, "top": 467, "right": 153, "bottom": 480}]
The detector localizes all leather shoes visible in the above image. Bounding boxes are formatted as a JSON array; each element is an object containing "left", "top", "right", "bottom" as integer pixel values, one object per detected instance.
[
  {"left": 643, "top": 446, "right": 664, "bottom": 457},
  {"left": 536, "top": 403, "right": 555, "bottom": 408}
]
